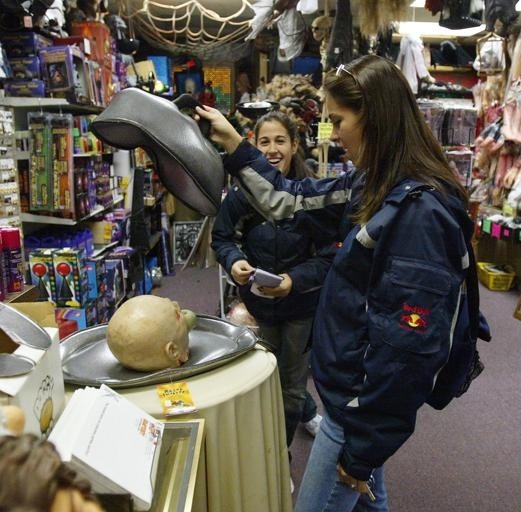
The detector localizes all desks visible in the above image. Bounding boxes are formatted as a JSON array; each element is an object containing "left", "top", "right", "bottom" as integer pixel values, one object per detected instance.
[{"left": 63, "top": 342, "right": 294, "bottom": 512}]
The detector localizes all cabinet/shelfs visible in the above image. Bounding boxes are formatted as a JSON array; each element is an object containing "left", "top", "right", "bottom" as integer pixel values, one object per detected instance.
[{"left": 3, "top": 95, "right": 125, "bottom": 229}]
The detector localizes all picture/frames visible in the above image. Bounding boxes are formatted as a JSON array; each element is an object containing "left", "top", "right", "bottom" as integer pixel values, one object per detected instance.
[
  {"left": 172, "top": 220, "right": 203, "bottom": 265},
  {"left": 174, "top": 71, "right": 204, "bottom": 98}
]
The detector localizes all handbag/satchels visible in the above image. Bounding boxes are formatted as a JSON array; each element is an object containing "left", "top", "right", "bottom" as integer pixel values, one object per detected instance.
[{"left": 426, "top": 292, "right": 492, "bottom": 410}]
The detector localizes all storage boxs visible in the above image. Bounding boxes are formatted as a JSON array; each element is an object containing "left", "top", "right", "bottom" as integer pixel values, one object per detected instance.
[{"left": 0, "top": 327, "right": 66, "bottom": 445}]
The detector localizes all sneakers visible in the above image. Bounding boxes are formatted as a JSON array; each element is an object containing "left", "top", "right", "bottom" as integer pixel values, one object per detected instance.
[{"left": 304, "top": 413, "right": 325, "bottom": 438}]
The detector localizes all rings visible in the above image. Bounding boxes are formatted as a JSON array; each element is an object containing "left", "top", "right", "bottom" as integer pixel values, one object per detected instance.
[{"left": 351, "top": 483, "right": 357, "bottom": 488}]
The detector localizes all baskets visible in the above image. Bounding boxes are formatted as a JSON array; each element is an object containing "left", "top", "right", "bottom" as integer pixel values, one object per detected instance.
[{"left": 474, "top": 261, "right": 518, "bottom": 292}]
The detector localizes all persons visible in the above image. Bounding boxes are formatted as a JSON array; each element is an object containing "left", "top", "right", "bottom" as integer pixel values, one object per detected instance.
[
  {"left": 209, "top": 110, "right": 344, "bottom": 493},
  {"left": 191, "top": 55, "right": 496, "bottom": 510}
]
